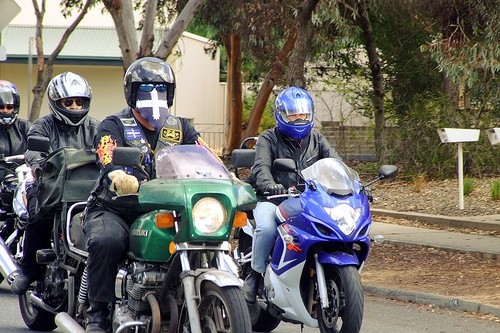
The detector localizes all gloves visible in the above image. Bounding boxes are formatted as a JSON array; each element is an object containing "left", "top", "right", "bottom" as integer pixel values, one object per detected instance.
[
  {"left": 361, "top": 187, "right": 373, "bottom": 203},
  {"left": 230, "top": 172, "right": 250, "bottom": 186},
  {"left": 108, "top": 169, "right": 139, "bottom": 196},
  {"left": 265, "top": 184, "right": 288, "bottom": 202}
]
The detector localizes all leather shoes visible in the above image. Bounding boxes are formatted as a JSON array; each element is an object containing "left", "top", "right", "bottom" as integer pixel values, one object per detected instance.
[
  {"left": 85, "top": 306, "right": 110, "bottom": 333},
  {"left": 244, "top": 270, "right": 261, "bottom": 306},
  {"left": 10, "top": 273, "right": 34, "bottom": 295}
]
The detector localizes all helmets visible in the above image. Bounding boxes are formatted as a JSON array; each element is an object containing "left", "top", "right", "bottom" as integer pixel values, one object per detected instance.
[
  {"left": 47, "top": 71, "right": 92, "bottom": 128},
  {"left": 0, "top": 80, "right": 20, "bottom": 126},
  {"left": 274, "top": 86, "right": 314, "bottom": 140},
  {"left": 124, "top": 57, "right": 176, "bottom": 109}
]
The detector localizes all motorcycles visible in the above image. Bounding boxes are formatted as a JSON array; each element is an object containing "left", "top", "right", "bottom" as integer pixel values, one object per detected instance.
[
  {"left": 0, "top": 149, "right": 85, "bottom": 332},
  {"left": 229, "top": 137, "right": 399, "bottom": 333},
  {"left": 35, "top": 144, "right": 259, "bottom": 333}
]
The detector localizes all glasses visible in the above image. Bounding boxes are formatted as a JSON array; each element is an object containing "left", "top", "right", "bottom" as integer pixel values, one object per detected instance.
[
  {"left": 58, "top": 98, "right": 84, "bottom": 107},
  {"left": 139, "top": 83, "right": 167, "bottom": 92},
  {"left": 0, "top": 105, "right": 13, "bottom": 110}
]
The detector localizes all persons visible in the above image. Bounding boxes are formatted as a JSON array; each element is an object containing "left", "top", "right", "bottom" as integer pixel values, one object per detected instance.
[
  {"left": 0, "top": 80, "right": 33, "bottom": 186},
  {"left": 24, "top": 70, "right": 101, "bottom": 292},
  {"left": 79, "top": 57, "right": 224, "bottom": 333},
  {"left": 243, "top": 86, "right": 372, "bottom": 304}
]
